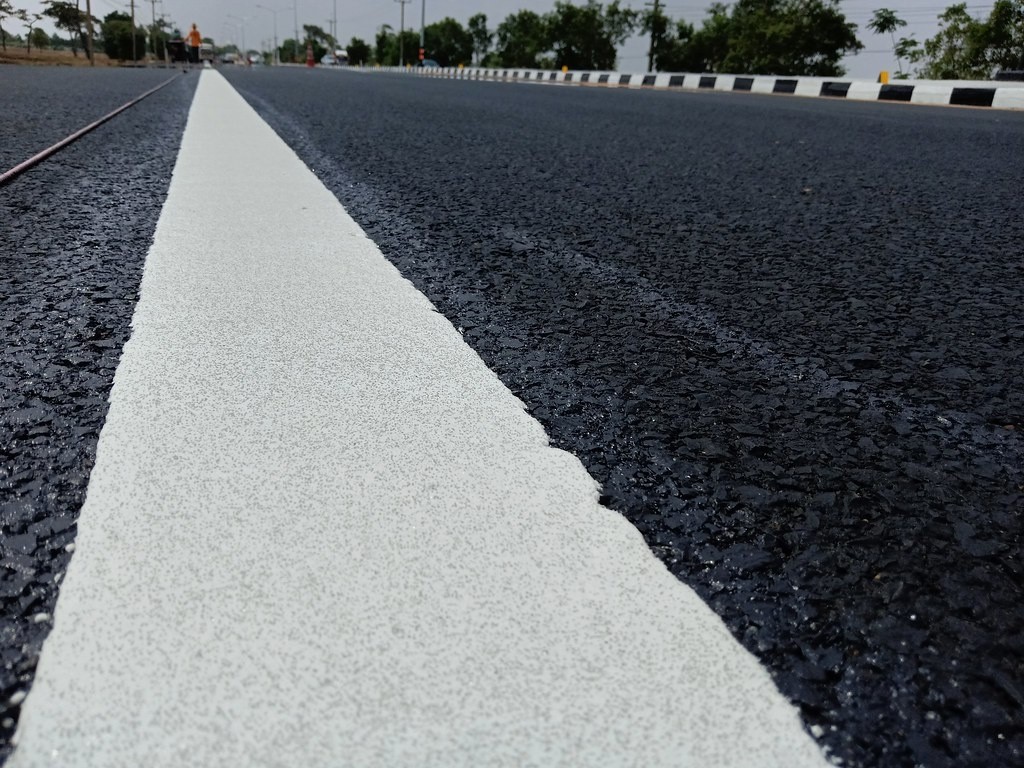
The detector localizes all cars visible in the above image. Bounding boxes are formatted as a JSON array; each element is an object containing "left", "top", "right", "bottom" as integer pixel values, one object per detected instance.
[
  {"left": 414, "top": 59, "right": 440, "bottom": 68},
  {"left": 219, "top": 50, "right": 263, "bottom": 67},
  {"left": 321, "top": 54, "right": 339, "bottom": 66},
  {"left": 996, "top": 70, "right": 1024, "bottom": 82}
]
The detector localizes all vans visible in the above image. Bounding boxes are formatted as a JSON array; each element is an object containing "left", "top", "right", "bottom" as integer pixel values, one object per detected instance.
[{"left": 197, "top": 43, "right": 214, "bottom": 64}]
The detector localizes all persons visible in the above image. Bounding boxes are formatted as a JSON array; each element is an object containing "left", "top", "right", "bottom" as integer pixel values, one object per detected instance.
[
  {"left": 182, "top": 23, "right": 202, "bottom": 63},
  {"left": 307, "top": 45, "right": 314, "bottom": 67}
]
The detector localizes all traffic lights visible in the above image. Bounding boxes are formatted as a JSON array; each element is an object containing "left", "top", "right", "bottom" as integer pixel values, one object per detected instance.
[{"left": 418, "top": 48, "right": 425, "bottom": 61}]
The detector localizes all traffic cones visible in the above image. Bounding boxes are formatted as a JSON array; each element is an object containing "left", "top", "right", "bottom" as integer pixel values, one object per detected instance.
[{"left": 306, "top": 40, "right": 316, "bottom": 68}]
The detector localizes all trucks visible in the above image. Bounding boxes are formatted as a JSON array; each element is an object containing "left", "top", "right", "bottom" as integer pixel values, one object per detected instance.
[{"left": 335, "top": 50, "right": 349, "bottom": 66}]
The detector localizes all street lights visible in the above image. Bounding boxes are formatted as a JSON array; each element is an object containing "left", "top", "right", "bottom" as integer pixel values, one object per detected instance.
[{"left": 255, "top": 5, "right": 293, "bottom": 67}]
